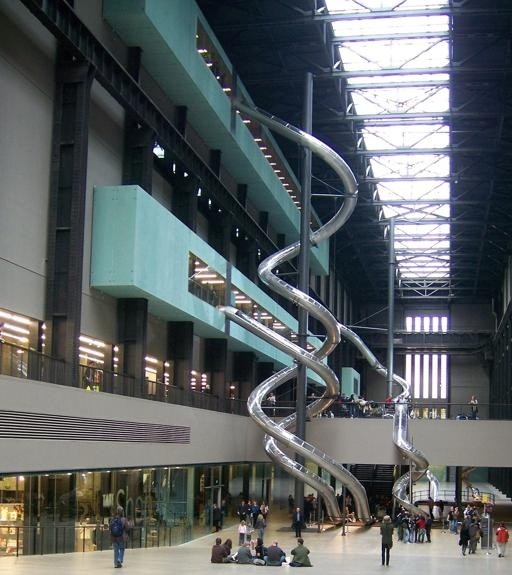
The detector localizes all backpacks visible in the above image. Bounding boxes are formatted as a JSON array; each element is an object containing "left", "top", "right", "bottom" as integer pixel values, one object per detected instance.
[{"left": 110, "top": 516, "right": 123, "bottom": 536}]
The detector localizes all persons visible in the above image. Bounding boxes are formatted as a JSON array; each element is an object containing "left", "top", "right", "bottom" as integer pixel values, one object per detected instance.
[
  {"left": 267, "top": 392, "right": 276, "bottom": 417},
  {"left": 462, "top": 467, "right": 477, "bottom": 501},
  {"left": 345, "top": 495, "right": 352, "bottom": 512},
  {"left": 110, "top": 506, "right": 135, "bottom": 568},
  {"left": 468, "top": 395, "right": 478, "bottom": 420},
  {"left": 376, "top": 497, "right": 444, "bottom": 543},
  {"left": 426, "top": 469, "right": 440, "bottom": 503},
  {"left": 381, "top": 515, "right": 393, "bottom": 566},
  {"left": 309, "top": 392, "right": 396, "bottom": 418},
  {"left": 81, "top": 361, "right": 97, "bottom": 391},
  {"left": 447, "top": 504, "right": 509, "bottom": 557},
  {"left": 211, "top": 491, "right": 316, "bottom": 567}
]
